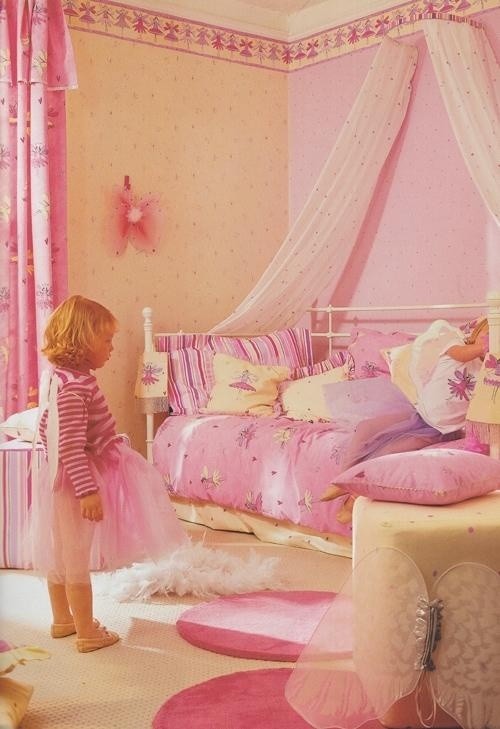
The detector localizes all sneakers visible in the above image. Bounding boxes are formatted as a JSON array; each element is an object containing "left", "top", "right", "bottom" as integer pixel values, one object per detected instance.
[
  {"left": 52, "top": 617, "right": 100, "bottom": 638},
  {"left": 76, "top": 631, "right": 119, "bottom": 652},
  {"left": 322, "top": 484, "right": 356, "bottom": 523}
]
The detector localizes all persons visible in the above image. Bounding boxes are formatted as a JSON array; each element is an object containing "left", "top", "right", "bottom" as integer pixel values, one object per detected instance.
[
  {"left": 38, "top": 296, "right": 134, "bottom": 655},
  {"left": 446, "top": 330, "right": 500, "bottom": 461}
]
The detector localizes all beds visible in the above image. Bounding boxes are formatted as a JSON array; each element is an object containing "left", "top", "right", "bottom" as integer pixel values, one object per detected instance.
[{"left": 136, "top": 289, "right": 500, "bottom": 552}]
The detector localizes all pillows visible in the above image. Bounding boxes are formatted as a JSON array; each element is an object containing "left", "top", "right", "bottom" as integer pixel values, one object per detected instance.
[
  {"left": 1, "top": 406, "right": 50, "bottom": 443},
  {"left": 1, "top": 637, "right": 52, "bottom": 676},
  {"left": 0, "top": 677, "right": 36, "bottom": 728},
  {"left": 331, "top": 447, "right": 500, "bottom": 506}
]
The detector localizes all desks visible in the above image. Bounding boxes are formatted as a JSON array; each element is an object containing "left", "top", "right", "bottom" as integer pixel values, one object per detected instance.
[
  {"left": 351, "top": 494, "right": 499, "bottom": 727},
  {"left": 1, "top": 437, "right": 45, "bottom": 569}
]
